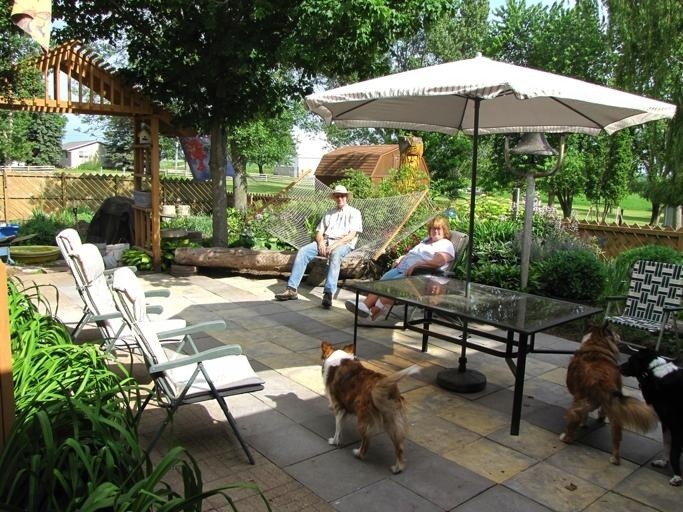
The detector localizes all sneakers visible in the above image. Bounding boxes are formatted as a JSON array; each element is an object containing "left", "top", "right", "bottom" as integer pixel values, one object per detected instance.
[
  {"left": 344, "top": 299, "right": 370, "bottom": 318},
  {"left": 274, "top": 286, "right": 298, "bottom": 301},
  {"left": 322, "top": 293, "right": 333, "bottom": 307}
]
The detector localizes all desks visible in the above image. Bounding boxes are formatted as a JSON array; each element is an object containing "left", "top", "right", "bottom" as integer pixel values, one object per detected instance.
[{"left": 337, "top": 273, "right": 603, "bottom": 436}]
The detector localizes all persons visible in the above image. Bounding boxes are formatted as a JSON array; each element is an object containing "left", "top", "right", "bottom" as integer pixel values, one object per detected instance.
[
  {"left": 275, "top": 185, "right": 362, "bottom": 306},
  {"left": 345, "top": 214, "right": 456, "bottom": 320}
]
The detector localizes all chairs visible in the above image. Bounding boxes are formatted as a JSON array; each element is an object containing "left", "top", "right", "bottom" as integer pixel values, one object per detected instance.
[
  {"left": 55, "top": 227, "right": 171, "bottom": 338},
  {"left": 604, "top": 259, "right": 683, "bottom": 362},
  {"left": 110, "top": 267, "right": 266, "bottom": 465},
  {"left": 68, "top": 242, "right": 186, "bottom": 354},
  {"left": 385, "top": 230, "right": 469, "bottom": 319}
]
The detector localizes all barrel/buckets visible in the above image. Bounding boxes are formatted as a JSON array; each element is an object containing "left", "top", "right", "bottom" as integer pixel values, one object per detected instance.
[{"left": 106, "top": 242, "right": 131, "bottom": 267}]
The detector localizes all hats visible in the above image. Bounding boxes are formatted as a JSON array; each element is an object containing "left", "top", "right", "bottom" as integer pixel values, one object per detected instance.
[{"left": 328, "top": 185, "right": 353, "bottom": 201}]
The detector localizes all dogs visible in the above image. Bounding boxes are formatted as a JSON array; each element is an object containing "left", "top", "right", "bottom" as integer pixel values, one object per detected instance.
[
  {"left": 619, "top": 346, "right": 683, "bottom": 487},
  {"left": 558, "top": 320, "right": 658, "bottom": 465},
  {"left": 321, "top": 340, "right": 432, "bottom": 475}
]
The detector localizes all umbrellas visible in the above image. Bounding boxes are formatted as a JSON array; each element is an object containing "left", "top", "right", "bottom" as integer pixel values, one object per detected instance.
[{"left": 303, "top": 52, "right": 677, "bottom": 370}]
